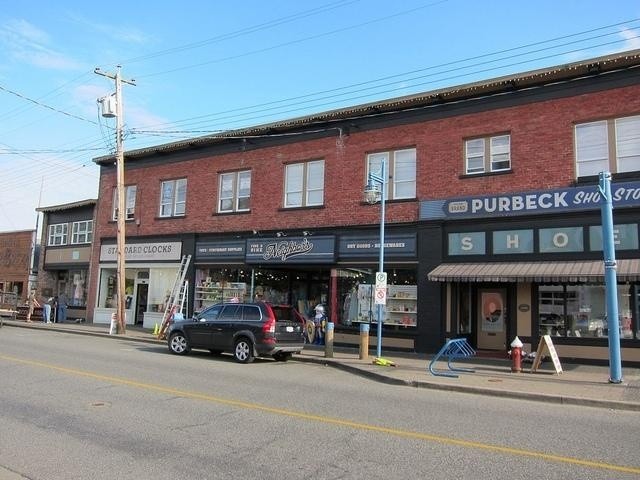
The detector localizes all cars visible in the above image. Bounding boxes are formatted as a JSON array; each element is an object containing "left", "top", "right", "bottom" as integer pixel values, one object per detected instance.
[{"left": 539, "top": 311, "right": 631, "bottom": 338}]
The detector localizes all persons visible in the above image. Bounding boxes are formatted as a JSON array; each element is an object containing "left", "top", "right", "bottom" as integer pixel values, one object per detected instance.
[
  {"left": 71, "top": 273, "right": 85, "bottom": 306},
  {"left": 57, "top": 292, "right": 68, "bottom": 323},
  {"left": 26, "top": 290, "right": 41, "bottom": 323},
  {"left": 313, "top": 304, "right": 326, "bottom": 345},
  {"left": 43, "top": 295, "right": 59, "bottom": 324}
]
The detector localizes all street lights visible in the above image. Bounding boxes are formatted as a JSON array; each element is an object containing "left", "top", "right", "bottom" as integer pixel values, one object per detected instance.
[{"left": 362, "top": 158, "right": 394, "bottom": 362}]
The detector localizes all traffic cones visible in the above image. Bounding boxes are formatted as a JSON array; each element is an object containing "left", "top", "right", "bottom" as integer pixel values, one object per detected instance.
[{"left": 152, "top": 322, "right": 160, "bottom": 336}]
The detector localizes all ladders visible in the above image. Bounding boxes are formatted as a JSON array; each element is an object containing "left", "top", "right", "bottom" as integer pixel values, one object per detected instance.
[{"left": 156, "top": 254, "right": 192, "bottom": 341}]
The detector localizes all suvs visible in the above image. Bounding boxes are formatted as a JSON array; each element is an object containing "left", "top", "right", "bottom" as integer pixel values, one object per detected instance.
[{"left": 165, "top": 300, "right": 309, "bottom": 365}]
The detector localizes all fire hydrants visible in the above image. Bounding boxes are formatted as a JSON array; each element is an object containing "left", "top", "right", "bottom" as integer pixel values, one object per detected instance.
[{"left": 507, "top": 336, "right": 524, "bottom": 374}]
